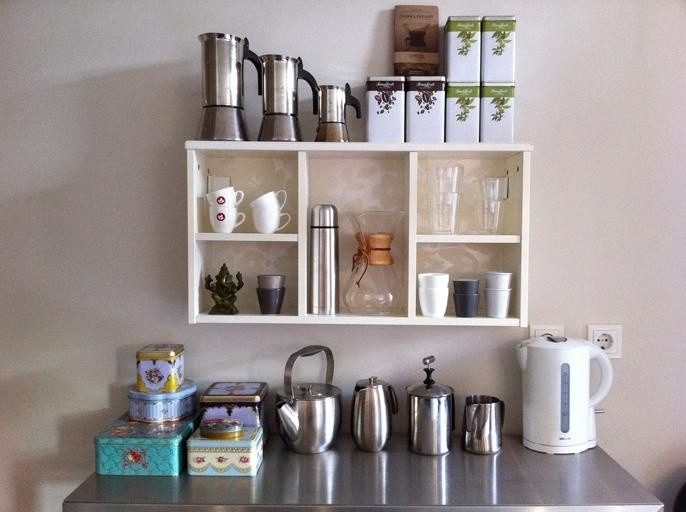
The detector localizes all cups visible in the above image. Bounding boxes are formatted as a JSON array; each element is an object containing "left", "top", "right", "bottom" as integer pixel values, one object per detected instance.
[
  {"left": 206, "top": 186, "right": 245, "bottom": 208},
  {"left": 452, "top": 279, "right": 479, "bottom": 295},
  {"left": 453, "top": 294, "right": 480, "bottom": 318},
  {"left": 252, "top": 211, "right": 291, "bottom": 235},
  {"left": 418, "top": 288, "right": 449, "bottom": 317},
  {"left": 249, "top": 189, "right": 288, "bottom": 211},
  {"left": 427, "top": 192, "right": 463, "bottom": 235},
  {"left": 483, "top": 288, "right": 513, "bottom": 319},
  {"left": 208, "top": 207, "right": 246, "bottom": 234},
  {"left": 484, "top": 271, "right": 513, "bottom": 289},
  {"left": 257, "top": 274, "right": 285, "bottom": 289},
  {"left": 417, "top": 273, "right": 450, "bottom": 288},
  {"left": 479, "top": 202, "right": 503, "bottom": 233},
  {"left": 256, "top": 287, "right": 286, "bottom": 315},
  {"left": 482, "top": 177, "right": 505, "bottom": 201},
  {"left": 427, "top": 164, "right": 464, "bottom": 192}
]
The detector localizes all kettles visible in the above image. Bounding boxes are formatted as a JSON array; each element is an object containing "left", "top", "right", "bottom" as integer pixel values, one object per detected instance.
[{"left": 516, "top": 335, "right": 613, "bottom": 457}]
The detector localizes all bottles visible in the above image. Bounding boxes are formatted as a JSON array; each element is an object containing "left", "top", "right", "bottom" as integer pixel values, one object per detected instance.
[{"left": 309, "top": 203, "right": 339, "bottom": 315}]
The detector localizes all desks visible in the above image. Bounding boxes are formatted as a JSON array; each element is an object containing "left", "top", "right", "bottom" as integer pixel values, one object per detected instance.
[{"left": 66, "top": 424, "right": 667, "bottom": 512}]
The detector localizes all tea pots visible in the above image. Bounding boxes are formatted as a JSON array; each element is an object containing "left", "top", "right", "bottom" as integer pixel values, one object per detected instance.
[
  {"left": 462, "top": 395, "right": 505, "bottom": 456},
  {"left": 404, "top": 356, "right": 455, "bottom": 456},
  {"left": 194, "top": 32, "right": 264, "bottom": 142},
  {"left": 272, "top": 344, "right": 342, "bottom": 454},
  {"left": 256, "top": 54, "right": 318, "bottom": 142},
  {"left": 315, "top": 82, "right": 362, "bottom": 143},
  {"left": 351, "top": 377, "right": 398, "bottom": 453}
]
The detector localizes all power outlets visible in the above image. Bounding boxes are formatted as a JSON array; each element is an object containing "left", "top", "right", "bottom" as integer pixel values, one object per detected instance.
[{"left": 587, "top": 325, "right": 623, "bottom": 358}]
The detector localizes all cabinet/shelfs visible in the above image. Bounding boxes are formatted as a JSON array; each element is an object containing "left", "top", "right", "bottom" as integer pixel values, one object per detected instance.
[{"left": 184, "top": 139, "right": 534, "bottom": 327}]
